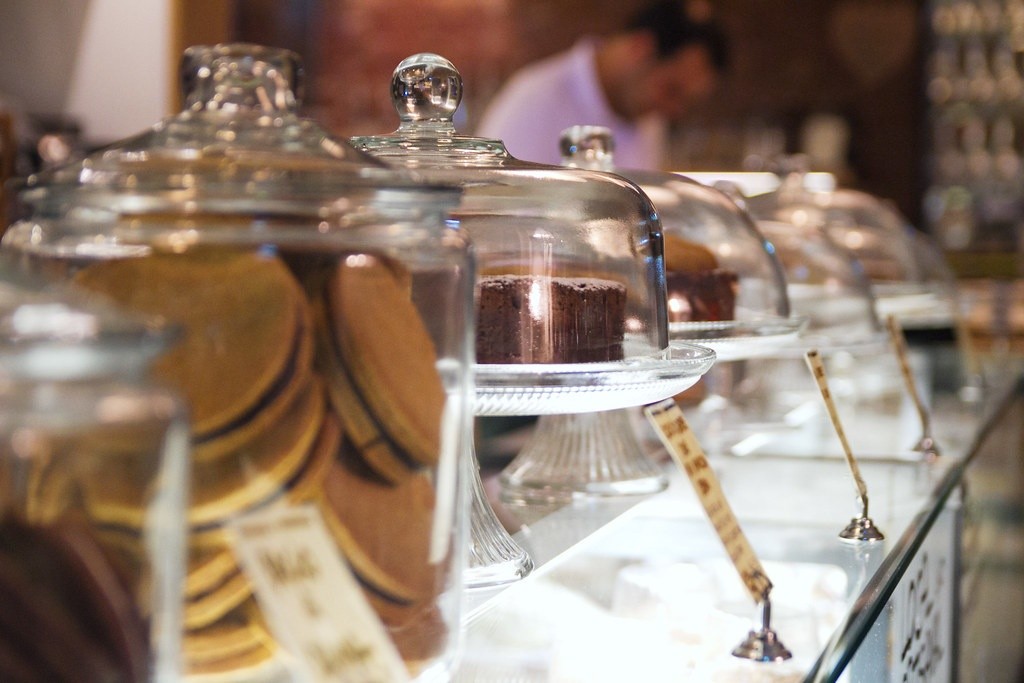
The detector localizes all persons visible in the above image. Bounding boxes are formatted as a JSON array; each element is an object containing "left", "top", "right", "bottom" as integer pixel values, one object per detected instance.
[{"left": 479, "top": 0, "right": 728, "bottom": 173}]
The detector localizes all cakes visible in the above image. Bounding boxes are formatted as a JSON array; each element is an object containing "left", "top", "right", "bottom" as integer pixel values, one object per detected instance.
[{"left": 475, "top": 236, "right": 740, "bottom": 363}]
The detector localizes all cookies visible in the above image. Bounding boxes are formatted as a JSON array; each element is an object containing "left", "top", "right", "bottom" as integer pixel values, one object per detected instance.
[{"left": 0, "top": 244, "right": 450, "bottom": 683}]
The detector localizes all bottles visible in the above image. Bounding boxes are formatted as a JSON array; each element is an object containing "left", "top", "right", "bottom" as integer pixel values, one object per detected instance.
[{"left": 0, "top": 40, "right": 971, "bottom": 682}]
